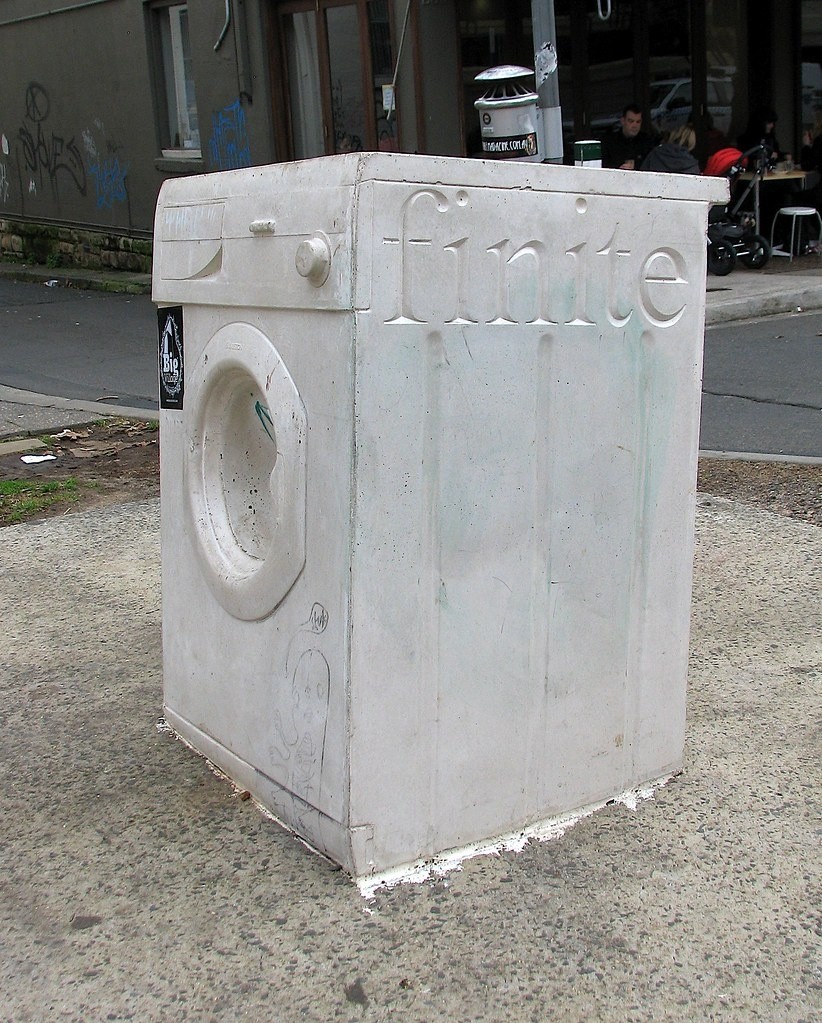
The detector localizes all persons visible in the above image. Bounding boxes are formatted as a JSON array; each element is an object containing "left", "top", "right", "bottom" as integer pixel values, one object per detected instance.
[
  {"left": 690, "top": 111, "right": 730, "bottom": 155},
  {"left": 640, "top": 125, "right": 699, "bottom": 173},
  {"left": 601, "top": 106, "right": 658, "bottom": 169},
  {"left": 748, "top": 109, "right": 792, "bottom": 162},
  {"left": 789, "top": 104, "right": 822, "bottom": 236}
]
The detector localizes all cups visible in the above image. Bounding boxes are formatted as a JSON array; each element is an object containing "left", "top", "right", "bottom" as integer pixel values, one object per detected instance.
[{"left": 784, "top": 161, "right": 794, "bottom": 172}]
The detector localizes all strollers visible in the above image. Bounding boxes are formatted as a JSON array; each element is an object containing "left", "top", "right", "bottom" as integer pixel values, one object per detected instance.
[{"left": 704, "top": 143, "right": 770, "bottom": 276}]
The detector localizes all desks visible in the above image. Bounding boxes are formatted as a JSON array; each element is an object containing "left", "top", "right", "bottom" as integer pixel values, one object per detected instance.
[{"left": 739, "top": 169, "right": 808, "bottom": 249}]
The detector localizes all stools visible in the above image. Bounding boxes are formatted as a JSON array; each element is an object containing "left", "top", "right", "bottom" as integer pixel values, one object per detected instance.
[{"left": 770, "top": 206, "right": 822, "bottom": 263}]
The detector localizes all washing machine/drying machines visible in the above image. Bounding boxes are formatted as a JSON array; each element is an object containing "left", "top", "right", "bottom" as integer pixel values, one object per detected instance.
[{"left": 151, "top": 150, "right": 731, "bottom": 876}]
